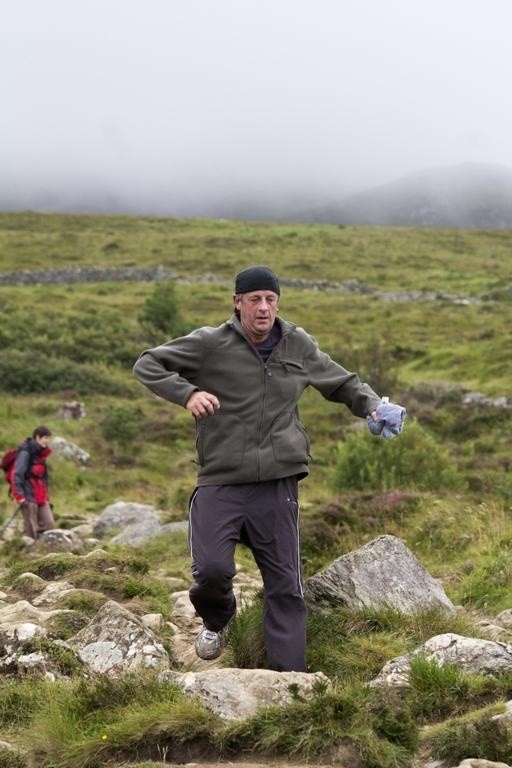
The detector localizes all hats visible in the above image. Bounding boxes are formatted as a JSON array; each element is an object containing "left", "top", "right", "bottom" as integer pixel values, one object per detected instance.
[{"left": 235, "top": 266, "right": 280, "bottom": 296}]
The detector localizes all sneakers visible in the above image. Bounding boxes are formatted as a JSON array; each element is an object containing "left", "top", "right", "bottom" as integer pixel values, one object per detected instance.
[{"left": 195, "top": 606, "right": 237, "bottom": 660}]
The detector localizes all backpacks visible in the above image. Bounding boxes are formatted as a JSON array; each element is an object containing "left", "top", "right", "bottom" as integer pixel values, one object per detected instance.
[{"left": 1, "top": 440, "right": 28, "bottom": 486}]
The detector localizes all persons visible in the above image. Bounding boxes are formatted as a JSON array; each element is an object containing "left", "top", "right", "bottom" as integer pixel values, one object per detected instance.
[
  {"left": 129, "top": 265, "right": 410, "bottom": 674},
  {"left": 6, "top": 425, "right": 56, "bottom": 536}
]
